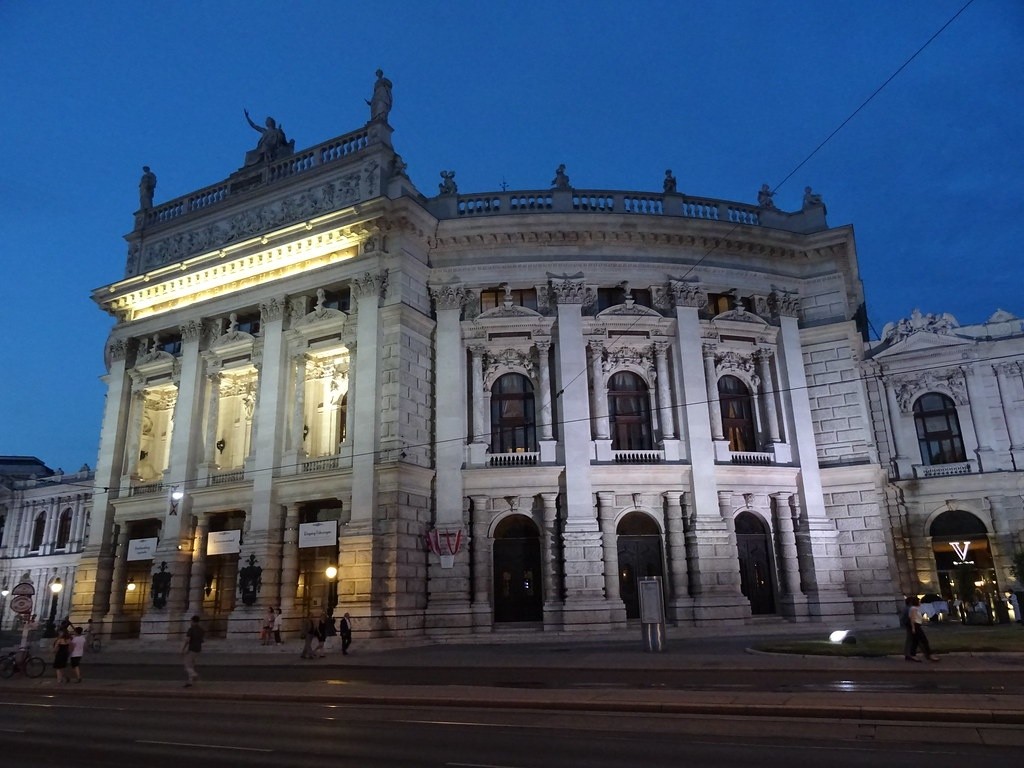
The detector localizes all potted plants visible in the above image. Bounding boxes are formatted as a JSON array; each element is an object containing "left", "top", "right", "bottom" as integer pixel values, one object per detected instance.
[{"left": 440, "top": 551, "right": 455, "bottom": 568}]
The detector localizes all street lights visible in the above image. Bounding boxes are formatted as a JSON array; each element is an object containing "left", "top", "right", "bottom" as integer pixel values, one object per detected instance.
[
  {"left": 326, "top": 566, "right": 337, "bottom": 612},
  {"left": 0, "top": 585, "right": 9, "bottom": 631},
  {"left": 43, "top": 575, "right": 63, "bottom": 638}
]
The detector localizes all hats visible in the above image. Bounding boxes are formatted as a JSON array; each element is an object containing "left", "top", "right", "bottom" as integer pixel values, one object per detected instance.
[
  {"left": 74, "top": 627, "right": 83, "bottom": 634},
  {"left": 190, "top": 615, "right": 200, "bottom": 622}
]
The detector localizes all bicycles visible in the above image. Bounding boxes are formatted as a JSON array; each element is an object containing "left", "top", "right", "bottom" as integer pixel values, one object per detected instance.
[
  {"left": 81, "top": 628, "right": 102, "bottom": 653},
  {"left": 0, "top": 644, "right": 46, "bottom": 678},
  {"left": 53, "top": 630, "right": 76, "bottom": 650}
]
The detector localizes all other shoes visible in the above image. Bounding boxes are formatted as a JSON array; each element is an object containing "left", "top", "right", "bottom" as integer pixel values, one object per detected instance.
[
  {"left": 343, "top": 652, "right": 348, "bottom": 655},
  {"left": 905, "top": 655, "right": 922, "bottom": 662},
  {"left": 193, "top": 673, "right": 198, "bottom": 681},
  {"left": 261, "top": 643, "right": 265, "bottom": 645},
  {"left": 66, "top": 677, "right": 70, "bottom": 683},
  {"left": 277, "top": 642, "right": 280, "bottom": 645},
  {"left": 319, "top": 655, "right": 325, "bottom": 657},
  {"left": 926, "top": 655, "right": 942, "bottom": 662},
  {"left": 182, "top": 684, "right": 192, "bottom": 688},
  {"left": 57, "top": 679, "right": 63, "bottom": 684},
  {"left": 312, "top": 650, "right": 316, "bottom": 656},
  {"left": 300, "top": 655, "right": 306, "bottom": 659},
  {"left": 77, "top": 677, "right": 81, "bottom": 683},
  {"left": 308, "top": 655, "right": 313, "bottom": 659}
]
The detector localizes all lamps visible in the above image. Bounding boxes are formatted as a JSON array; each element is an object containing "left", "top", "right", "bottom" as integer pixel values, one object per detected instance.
[
  {"left": 304, "top": 426, "right": 308, "bottom": 442},
  {"left": 261, "top": 236, "right": 269, "bottom": 245},
  {"left": 109, "top": 286, "right": 115, "bottom": 293},
  {"left": 219, "top": 250, "right": 226, "bottom": 258},
  {"left": 128, "top": 576, "right": 137, "bottom": 592},
  {"left": 143, "top": 274, "right": 150, "bottom": 283},
  {"left": 326, "top": 562, "right": 337, "bottom": 583},
  {"left": 180, "top": 262, "right": 186, "bottom": 271},
  {"left": 216, "top": 439, "right": 225, "bottom": 454},
  {"left": 306, "top": 221, "right": 313, "bottom": 230},
  {"left": 353, "top": 205, "right": 362, "bottom": 216}
]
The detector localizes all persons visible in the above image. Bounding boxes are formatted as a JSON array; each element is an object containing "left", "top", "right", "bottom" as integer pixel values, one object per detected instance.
[
  {"left": 802, "top": 186, "right": 822, "bottom": 210},
  {"left": 663, "top": 169, "right": 676, "bottom": 193},
  {"left": 137, "top": 166, "right": 157, "bottom": 210},
  {"left": 942, "top": 588, "right": 1022, "bottom": 625},
  {"left": 180, "top": 615, "right": 205, "bottom": 688},
  {"left": 551, "top": 163, "right": 570, "bottom": 189},
  {"left": 438, "top": 170, "right": 458, "bottom": 194},
  {"left": 904, "top": 596, "right": 940, "bottom": 662},
  {"left": 758, "top": 183, "right": 777, "bottom": 208},
  {"left": 17, "top": 614, "right": 94, "bottom": 684},
  {"left": 364, "top": 69, "right": 393, "bottom": 124},
  {"left": 301, "top": 611, "right": 352, "bottom": 660},
  {"left": 258, "top": 606, "right": 283, "bottom": 646},
  {"left": 244, "top": 108, "right": 288, "bottom": 162}
]
